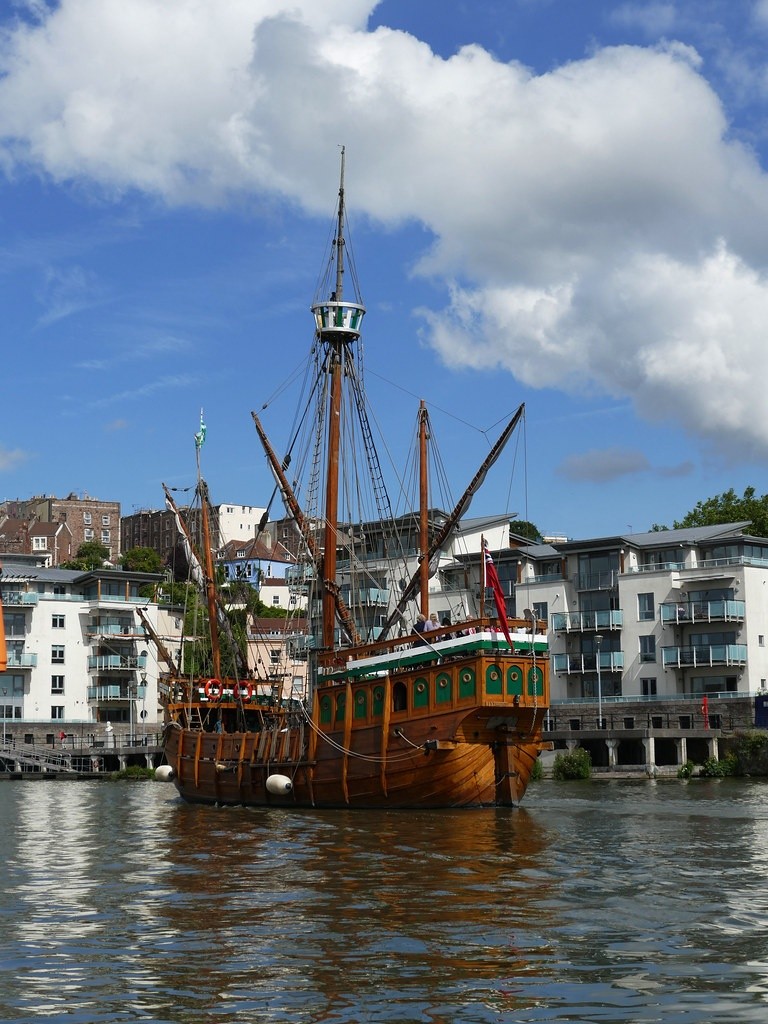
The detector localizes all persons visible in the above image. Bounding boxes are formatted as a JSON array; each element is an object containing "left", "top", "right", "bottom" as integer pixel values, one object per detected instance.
[
  {"left": 105, "top": 721, "right": 114, "bottom": 732},
  {"left": 424, "top": 613, "right": 443, "bottom": 642},
  {"left": 441, "top": 616, "right": 543, "bottom": 638},
  {"left": 58, "top": 730, "right": 67, "bottom": 750},
  {"left": 411, "top": 614, "right": 428, "bottom": 648}
]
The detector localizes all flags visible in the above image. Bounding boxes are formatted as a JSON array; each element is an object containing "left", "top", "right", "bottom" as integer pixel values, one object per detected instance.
[
  {"left": 194, "top": 406, "right": 206, "bottom": 447},
  {"left": 482, "top": 536, "right": 512, "bottom": 654}
]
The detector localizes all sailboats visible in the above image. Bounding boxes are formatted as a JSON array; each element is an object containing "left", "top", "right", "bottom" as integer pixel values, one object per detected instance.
[{"left": 136, "top": 144, "right": 551, "bottom": 812}]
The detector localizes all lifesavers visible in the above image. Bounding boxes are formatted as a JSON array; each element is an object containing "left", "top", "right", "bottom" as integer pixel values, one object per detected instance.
[
  {"left": 205, "top": 679, "right": 223, "bottom": 700},
  {"left": 234, "top": 681, "right": 252, "bottom": 702}
]
